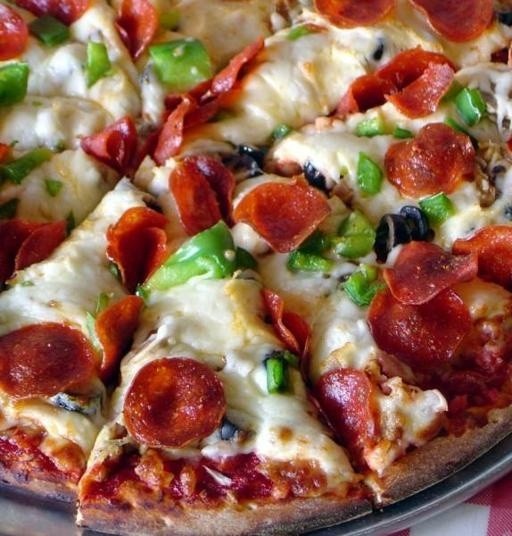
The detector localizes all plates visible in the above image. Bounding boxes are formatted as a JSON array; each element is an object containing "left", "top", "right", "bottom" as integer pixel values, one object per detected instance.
[{"left": 3, "top": 389, "right": 512, "bottom": 536}]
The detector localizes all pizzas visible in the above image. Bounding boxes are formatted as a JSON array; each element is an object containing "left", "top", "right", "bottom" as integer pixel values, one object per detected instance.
[{"left": 0, "top": 1, "right": 512, "bottom": 535}]
